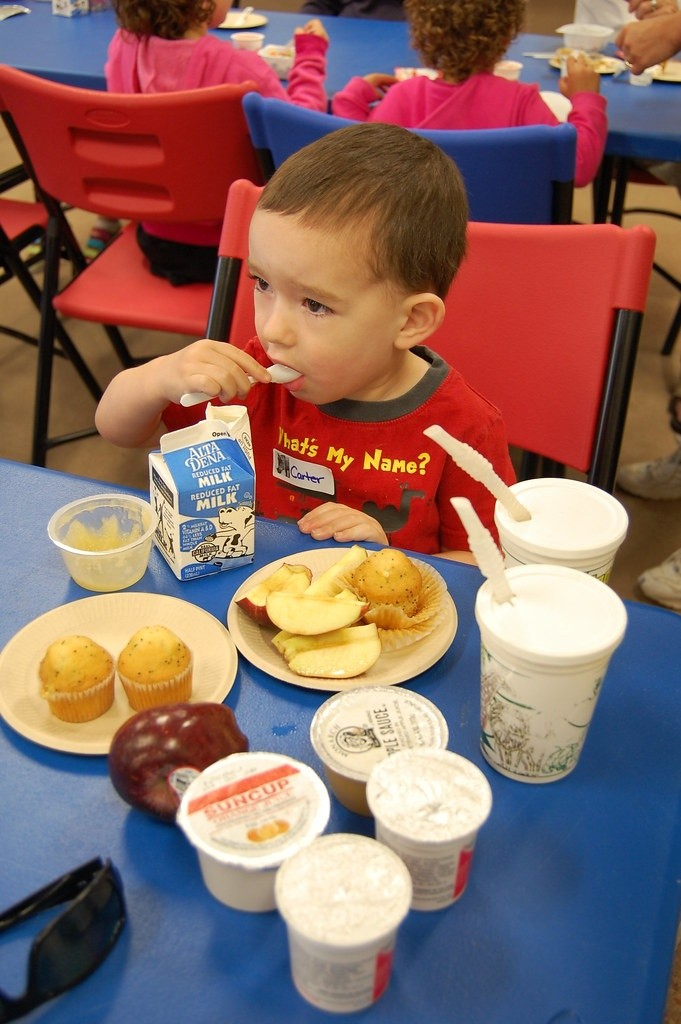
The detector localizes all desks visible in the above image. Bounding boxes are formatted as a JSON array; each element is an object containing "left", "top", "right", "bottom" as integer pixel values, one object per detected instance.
[
  {"left": 0, "top": 0, "right": 681, "bottom": 226},
  {"left": 0, "top": 459, "right": 680, "bottom": 1024}
]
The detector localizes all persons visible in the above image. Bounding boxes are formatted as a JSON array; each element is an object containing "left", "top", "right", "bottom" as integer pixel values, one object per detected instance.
[
  {"left": 95, "top": 122, "right": 518, "bottom": 565},
  {"left": 615, "top": 0, "right": 681, "bottom": 610},
  {"left": 332, "top": 0, "right": 608, "bottom": 187},
  {"left": 106, "top": 0, "right": 329, "bottom": 286}
]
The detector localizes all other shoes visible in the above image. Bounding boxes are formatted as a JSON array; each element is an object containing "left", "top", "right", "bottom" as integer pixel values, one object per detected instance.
[
  {"left": 84, "top": 226, "right": 112, "bottom": 263},
  {"left": 616, "top": 448, "right": 681, "bottom": 501},
  {"left": 640, "top": 549, "right": 681, "bottom": 609}
]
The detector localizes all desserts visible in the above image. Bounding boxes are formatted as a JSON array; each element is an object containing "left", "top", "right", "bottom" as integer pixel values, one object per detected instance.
[
  {"left": 354, "top": 548, "right": 423, "bottom": 618},
  {"left": 39, "top": 636, "right": 116, "bottom": 723},
  {"left": 117, "top": 624, "right": 194, "bottom": 712}
]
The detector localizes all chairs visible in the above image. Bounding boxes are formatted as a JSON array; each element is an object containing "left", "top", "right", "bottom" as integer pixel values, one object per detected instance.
[{"left": 0, "top": 62, "right": 657, "bottom": 491}]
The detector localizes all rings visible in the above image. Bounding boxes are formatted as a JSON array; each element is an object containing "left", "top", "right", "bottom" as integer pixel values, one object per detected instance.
[{"left": 651, "top": 0, "right": 656, "bottom": 6}]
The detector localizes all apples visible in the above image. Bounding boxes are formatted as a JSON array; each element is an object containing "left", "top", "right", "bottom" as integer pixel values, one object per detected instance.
[
  {"left": 107, "top": 703, "right": 248, "bottom": 821},
  {"left": 234, "top": 545, "right": 381, "bottom": 678}
]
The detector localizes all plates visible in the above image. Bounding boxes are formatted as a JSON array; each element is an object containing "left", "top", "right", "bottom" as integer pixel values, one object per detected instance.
[
  {"left": 1, "top": 593, "right": 239, "bottom": 756},
  {"left": 226, "top": 548, "right": 461, "bottom": 693},
  {"left": 550, "top": 54, "right": 629, "bottom": 74},
  {"left": 538, "top": 91, "right": 573, "bottom": 123},
  {"left": 647, "top": 59, "right": 680, "bottom": 83},
  {"left": 216, "top": 11, "right": 268, "bottom": 28}
]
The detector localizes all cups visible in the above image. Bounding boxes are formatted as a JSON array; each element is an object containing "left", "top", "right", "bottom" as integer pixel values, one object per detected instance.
[
  {"left": 494, "top": 476, "right": 629, "bottom": 583},
  {"left": 555, "top": 23, "right": 616, "bottom": 53},
  {"left": 47, "top": 492, "right": 159, "bottom": 592},
  {"left": 475, "top": 563, "right": 628, "bottom": 785},
  {"left": 365, "top": 748, "right": 493, "bottom": 912},
  {"left": 174, "top": 750, "right": 330, "bottom": 911},
  {"left": 493, "top": 60, "right": 524, "bottom": 82},
  {"left": 231, "top": 32, "right": 265, "bottom": 52},
  {"left": 309, "top": 683, "right": 450, "bottom": 820},
  {"left": 257, "top": 43, "right": 296, "bottom": 80},
  {"left": 275, "top": 832, "right": 414, "bottom": 1015}
]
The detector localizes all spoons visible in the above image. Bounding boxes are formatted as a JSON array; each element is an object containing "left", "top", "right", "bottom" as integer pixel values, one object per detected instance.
[{"left": 180, "top": 362, "right": 303, "bottom": 408}]
